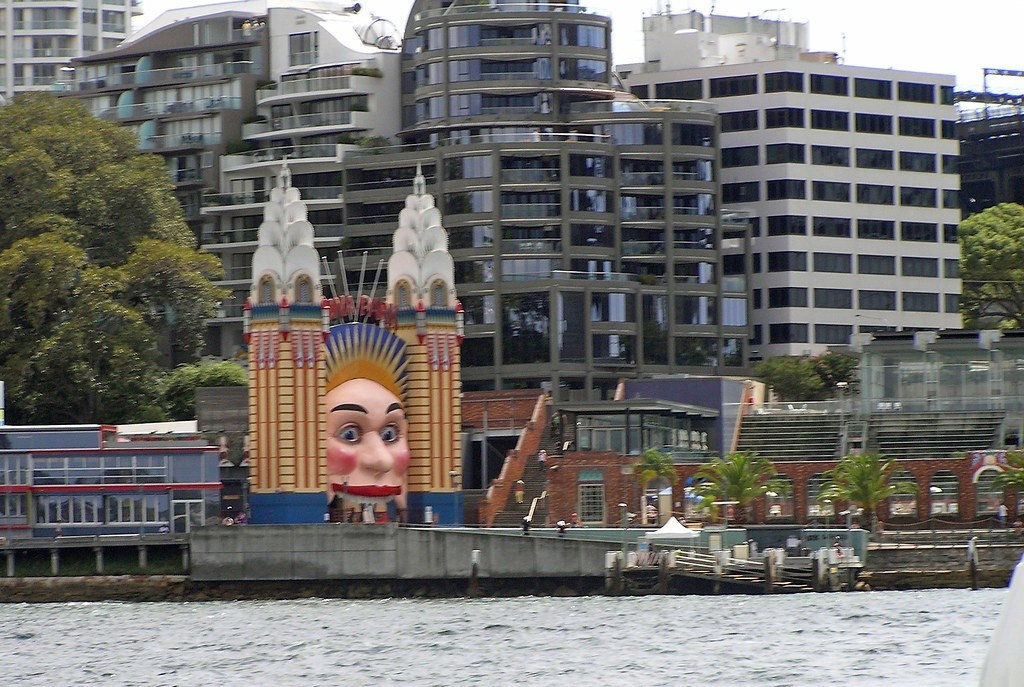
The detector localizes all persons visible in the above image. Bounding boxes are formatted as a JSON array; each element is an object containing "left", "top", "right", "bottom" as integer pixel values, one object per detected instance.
[
  {"left": 544, "top": 390, "right": 568, "bottom": 454},
  {"left": 646, "top": 502, "right": 658, "bottom": 524},
  {"left": 556, "top": 520, "right": 567, "bottom": 538},
  {"left": 726, "top": 506, "right": 884, "bottom": 558},
  {"left": 323, "top": 507, "right": 361, "bottom": 524},
  {"left": 538, "top": 449, "right": 547, "bottom": 471},
  {"left": 747, "top": 395, "right": 754, "bottom": 414},
  {"left": 233, "top": 510, "right": 247, "bottom": 525},
  {"left": 520, "top": 516, "right": 532, "bottom": 535},
  {"left": 570, "top": 513, "right": 581, "bottom": 528},
  {"left": 998, "top": 503, "right": 1022, "bottom": 541},
  {"left": 514, "top": 480, "right": 525, "bottom": 503}
]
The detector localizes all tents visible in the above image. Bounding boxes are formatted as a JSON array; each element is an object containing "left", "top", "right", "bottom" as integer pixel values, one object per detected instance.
[{"left": 645, "top": 517, "right": 702, "bottom": 553}]
